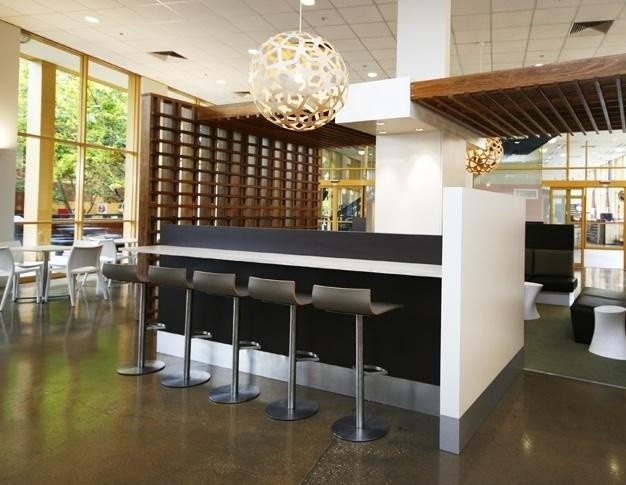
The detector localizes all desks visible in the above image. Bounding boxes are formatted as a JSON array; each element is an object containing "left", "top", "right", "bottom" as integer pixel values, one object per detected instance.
[
  {"left": 525, "top": 281, "right": 543, "bottom": 321},
  {"left": 589, "top": 306, "right": 626, "bottom": 360}
]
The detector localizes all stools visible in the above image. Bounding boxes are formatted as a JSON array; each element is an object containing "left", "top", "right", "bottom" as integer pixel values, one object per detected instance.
[{"left": 570, "top": 286, "right": 626, "bottom": 344}]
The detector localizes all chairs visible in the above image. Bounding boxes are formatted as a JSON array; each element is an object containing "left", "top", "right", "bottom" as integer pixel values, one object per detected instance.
[{"left": 0, "top": 239, "right": 131, "bottom": 311}]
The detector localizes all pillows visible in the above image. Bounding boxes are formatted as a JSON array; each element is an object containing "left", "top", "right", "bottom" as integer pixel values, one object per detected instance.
[
  {"left": 525, "top": 249, "right": 534, "bottom": 275},
  {"left": 535, "top": 250, "right": 574, "bottom": 277}
]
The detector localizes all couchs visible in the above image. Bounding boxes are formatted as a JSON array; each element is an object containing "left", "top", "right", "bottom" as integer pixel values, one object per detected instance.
[{"left": 526, "top": 224, "right": 581, "bottom": 306}]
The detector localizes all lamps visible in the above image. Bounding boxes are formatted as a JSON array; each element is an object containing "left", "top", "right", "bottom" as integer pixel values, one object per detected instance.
[
  {"left": 467, "top": 136, "right": 503, "bottom": 175},
  {"left": 249, "top": 0, "right": 350, "bottom": 131}
]
